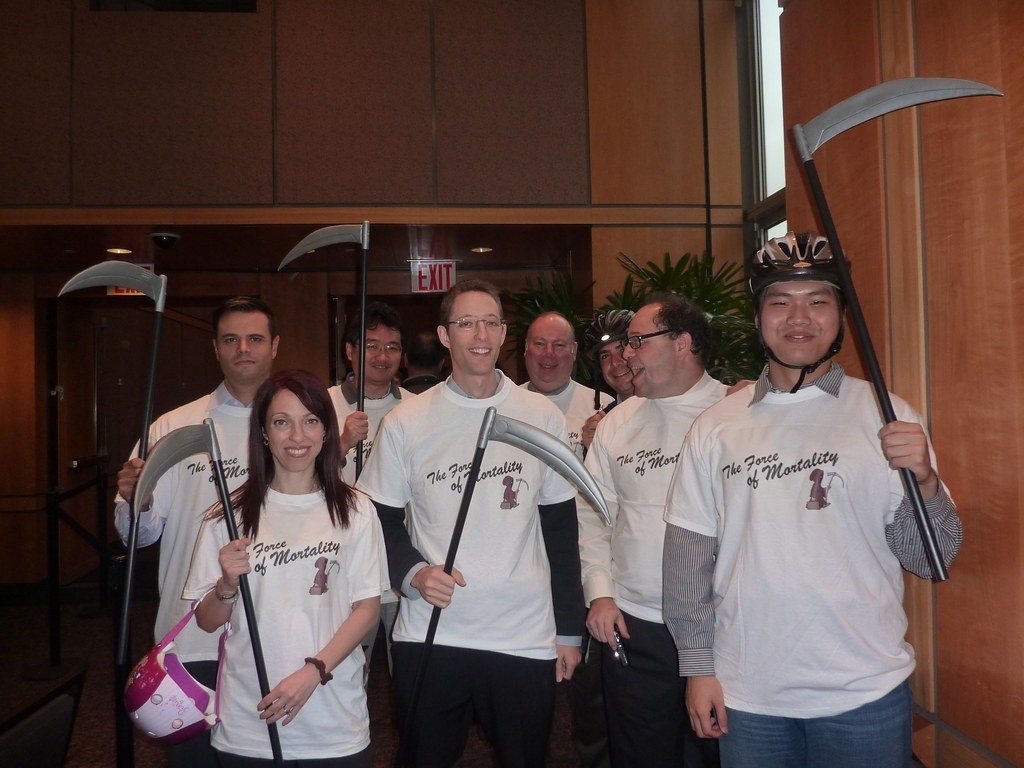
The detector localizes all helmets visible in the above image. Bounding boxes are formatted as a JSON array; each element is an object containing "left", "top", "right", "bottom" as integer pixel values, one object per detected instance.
[
  {"left": 123, "top": 641, "right": 217, "bottom": 746},
  {"left": 746, "top": 231, "right": 852, "bottom": 311},
  {"left": 583, "top": 310, "right": 636, "bottom": 361}
]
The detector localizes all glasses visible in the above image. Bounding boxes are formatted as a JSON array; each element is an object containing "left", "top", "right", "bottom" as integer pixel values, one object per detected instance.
[
  {"left": 621, "top": 329, "right": 670, "bottom": 349},
  {"left": 366, "top": 342, "right": 401, "bottom": 354},
  {"left": 448, "top": 315, "right": 500, "bottom": 330}
]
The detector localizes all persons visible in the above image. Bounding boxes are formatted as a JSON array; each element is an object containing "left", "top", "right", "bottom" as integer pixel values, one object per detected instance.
[
  {"left": 113, "top": 293, "right": 393, "bottom": 767},
  {"left": 325, "top": 230, "right": 952, "bottom": 768}
]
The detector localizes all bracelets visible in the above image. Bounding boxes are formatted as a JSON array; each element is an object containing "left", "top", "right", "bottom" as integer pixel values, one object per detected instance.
[{"left": 302, "top": 656, "right": 336, "bottom": 686}]
